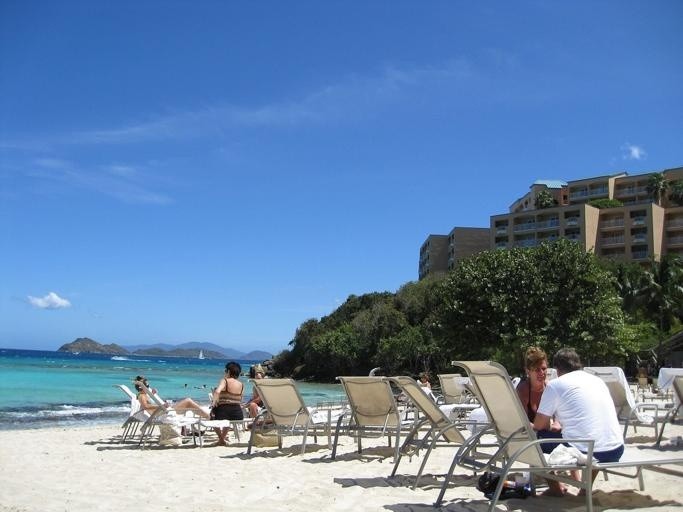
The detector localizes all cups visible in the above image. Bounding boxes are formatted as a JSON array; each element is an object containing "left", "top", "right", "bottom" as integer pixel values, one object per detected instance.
[
  {"left": 167, "top": 400, "right": 174, "bottom": 408},
  {"left": 515, "top": 474, "right": 526, "bottom": 489}
]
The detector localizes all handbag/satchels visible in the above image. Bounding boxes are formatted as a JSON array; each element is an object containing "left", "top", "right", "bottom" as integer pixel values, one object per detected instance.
[{"left": 250, "top": 429, "right": 284, "bottom": 447}]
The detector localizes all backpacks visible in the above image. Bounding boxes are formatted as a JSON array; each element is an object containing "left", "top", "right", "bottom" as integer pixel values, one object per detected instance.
[{"left": 477, "top": 472, "right": 532, "bottom": 500}]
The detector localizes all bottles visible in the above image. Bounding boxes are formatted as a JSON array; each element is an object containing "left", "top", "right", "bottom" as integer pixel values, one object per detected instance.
[{"left": 503, "top": 479, "right": 526, "bottom": 489}]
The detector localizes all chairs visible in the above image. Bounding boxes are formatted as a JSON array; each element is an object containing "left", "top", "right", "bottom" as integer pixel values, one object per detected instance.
[
  {"left": 330, "top": 361, "right": 682, "bottom": 511},
  {"left": 120, "top": 381, "right": 334, "bottom": 456}
]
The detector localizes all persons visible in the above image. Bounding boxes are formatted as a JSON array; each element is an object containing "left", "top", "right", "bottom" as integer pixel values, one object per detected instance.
[
  {"left": 239, "top": 371, "right": 274, "bottom": 432},
  {"left": 132, "top": 373, "right": 210, "bottom": 422},
  {"left": 513, "top": 346, "right": 562, "bottom": 432},
  {"left": 208, "top": 360, "right": 244, "bottom": 447},
  {"left": 208, "top": 386, "right": 218, "bottom": 409},
  {"left": 529, "top": 347, "right": 624, "bottom": 497},
  {"left": 393, "top": 372, "right": 431, "bottom": 403}
]
